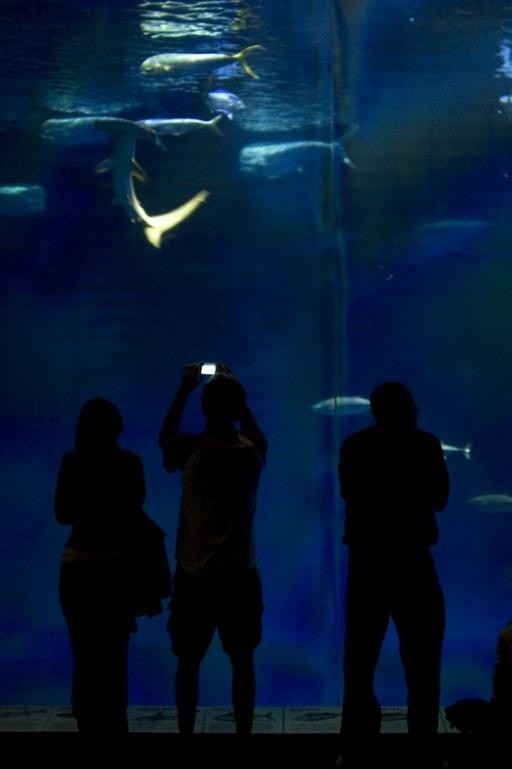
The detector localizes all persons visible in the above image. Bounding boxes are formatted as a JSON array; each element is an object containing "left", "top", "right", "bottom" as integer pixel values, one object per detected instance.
[
  {"left": 53, "top": 396, "right": 146, "bottom": 769},
  {"left": 158, "top": 361, "right": 268, "bottom": 767},
  {"left": 337, "top": 380, "right": 451, "bottom": 768}
]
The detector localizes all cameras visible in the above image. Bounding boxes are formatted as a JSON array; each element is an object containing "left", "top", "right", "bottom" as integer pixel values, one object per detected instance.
[{"left": 200, "top": 364, "right": 216, "bottom": 375}]
